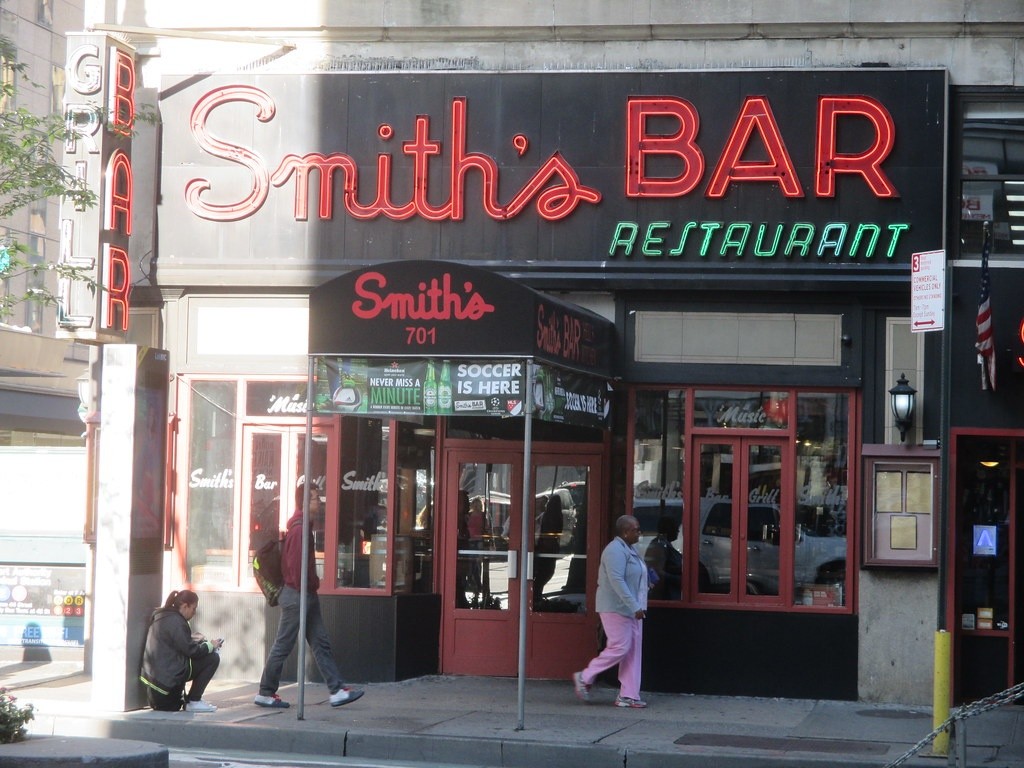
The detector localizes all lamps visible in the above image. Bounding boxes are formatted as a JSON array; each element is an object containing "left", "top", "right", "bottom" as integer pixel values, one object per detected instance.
[{"left": 889, "top": 374, "right": 918, "bottom": 441}]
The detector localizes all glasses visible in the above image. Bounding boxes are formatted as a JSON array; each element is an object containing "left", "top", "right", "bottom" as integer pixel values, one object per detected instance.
[{"left": 311, "top": 495, "right": 321, "bottom": 501}]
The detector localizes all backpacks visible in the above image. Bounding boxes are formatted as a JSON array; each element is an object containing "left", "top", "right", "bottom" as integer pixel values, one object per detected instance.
[{"left": 253, "top": 519, "right": 314, "bottom": 606}]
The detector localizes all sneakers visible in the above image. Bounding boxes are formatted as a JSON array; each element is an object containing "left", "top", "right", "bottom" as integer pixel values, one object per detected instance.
[
  {"left": 574, "top": 672, "right": 592, "bottom": 701},
  {"left": 329, "top": 687, "right": 365, "bottom": 707},
  {"left": 615, "top": 694, "right": 647, "bottom": 707},
  {"left": 255, "top": 694, "right": 291, "bottom": 708},
  {"left": 186, "top": 699, "right": 217, "bottom": 712}
]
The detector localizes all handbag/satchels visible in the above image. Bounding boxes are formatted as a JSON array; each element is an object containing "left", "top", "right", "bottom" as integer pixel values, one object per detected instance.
[{"left": 647, "top": 567, "right": 659, "bottom": 591}]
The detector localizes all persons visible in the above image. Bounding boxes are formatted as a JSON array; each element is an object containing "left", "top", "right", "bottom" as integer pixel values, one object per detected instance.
[
  {"left": 645, "top": 515, "right": 682, "bottom": 602},
  {"left": 573, "top": 514, "right": 654, "bottom": 708},
  {"left": 139, "top": 590, "right": 224, "bottom": 711},
  {"left": 253, "top": 481, "right": 366, "bottom": 707},
  {"left": 416, "top": 489, "right": 547, "bottom": 593}
]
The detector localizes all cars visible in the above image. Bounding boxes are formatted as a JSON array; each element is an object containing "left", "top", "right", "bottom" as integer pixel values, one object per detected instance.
[
  {"left": 481, "top": 481, "right": 586, "bottom": 546},
  {"left": 632, "top": 497, "right": 847, "bottom": 594}
]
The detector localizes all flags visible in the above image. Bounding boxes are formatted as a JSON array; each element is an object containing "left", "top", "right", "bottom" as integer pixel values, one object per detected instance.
[{"left": 974, "top": 232, "right": 998, "bottom": 393}]
